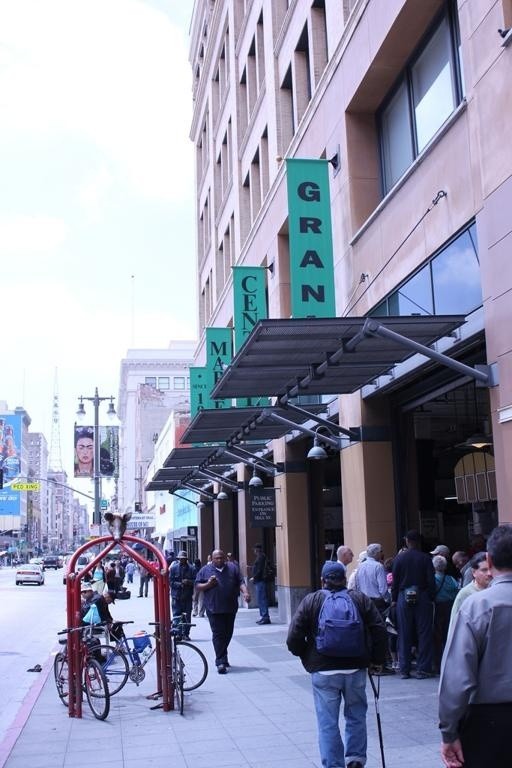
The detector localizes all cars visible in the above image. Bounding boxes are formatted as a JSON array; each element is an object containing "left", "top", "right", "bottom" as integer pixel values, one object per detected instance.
[
  {"left": 28, "top": 552, "right": 93, "bottom": 570},
  {"left": 16, "top": 564, "right": 45, "bottom": 586}
]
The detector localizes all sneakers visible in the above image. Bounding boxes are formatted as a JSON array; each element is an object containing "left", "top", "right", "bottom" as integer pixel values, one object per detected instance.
[
  {"left": 346, "top": 760, "right": 363, "bottom": 768},
  {"left": 216, "top": 662, "right": 231, "bottom": 674},
  {"left": 368, "top": 660, "right": 440, "bottom": 680},
  {"left": 173, "top": 634, "right": 191, "bottom": 642}
]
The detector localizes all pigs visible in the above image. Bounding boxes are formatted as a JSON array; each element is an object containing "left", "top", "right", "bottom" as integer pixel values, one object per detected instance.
[{"left": 103, "top": 510, "right": 133, "bottom": 542}]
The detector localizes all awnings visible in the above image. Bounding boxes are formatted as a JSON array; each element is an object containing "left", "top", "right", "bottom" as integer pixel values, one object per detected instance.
[{"left": 132, "top": 543, "right": 144, "bottom": 550}]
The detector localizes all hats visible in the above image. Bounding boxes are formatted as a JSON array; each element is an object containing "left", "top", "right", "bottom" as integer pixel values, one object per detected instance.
[
  {"left": 174, "top": 550, "right": 188, "bottom": 559},
  {"left": 107, "top": 591, "right": 116, "bottom": 605},
  {"left": 429, "top": 545, "right": 449, "bottom": 555},
  {"left": 80, "top": 582, "right": 93, "bottom": 593},
  {"left": 320, "top": 560, "right": 345, "bottom": 580}
]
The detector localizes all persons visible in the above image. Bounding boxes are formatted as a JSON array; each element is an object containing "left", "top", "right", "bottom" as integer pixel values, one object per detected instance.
[
  {"left": 338, "top": 533, "right": 494, "bottom": 680},
  {"left": 74, "top": 431, "right": 94, "bottom": 474},
  {"left": 286, "top": 561, "right": 388, "bottom": 768},
  {"left": 166, "top": 543, "right": 271, "bottom": 674},
  {"left": 3, "top": 554, "right": 7, "bottom": 566},
  {"left": 57, "top": 556, "right": 159, "bottom": 662},
  {"left": 438, "top": 525, "right": 512, "bottom": 768}
]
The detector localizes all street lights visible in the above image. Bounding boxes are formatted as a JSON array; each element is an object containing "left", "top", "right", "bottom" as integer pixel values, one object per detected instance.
[{"left": 76, "top": 386, "right": 117, "bottom": 558}]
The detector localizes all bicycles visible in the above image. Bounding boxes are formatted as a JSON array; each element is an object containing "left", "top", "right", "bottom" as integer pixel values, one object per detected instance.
[
  {"left": 54, "top": 622, "right": 110, "bottom": 720},
  {"left": 80, "top": 612, "right": 208, "bottom": 698},
  {"left": 149, "top": 620, "right": 196, "bottom": 715}
]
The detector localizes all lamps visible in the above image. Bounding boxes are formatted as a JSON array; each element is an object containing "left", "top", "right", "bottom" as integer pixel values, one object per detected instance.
[
  {"left": 461, "top": 377, "right": 493, "bottom": 448},
  {"left": 248, "top": 461, "right": 282, "bottom": 487},
  {"left": 195, "top": 500, "right": 206, "bottom": 509},
  {"left": 305, "top": 424, "right": 358, "bottom": 461},
  {"left": 216, "top": 480, "right": 244, "bottom": 500}
]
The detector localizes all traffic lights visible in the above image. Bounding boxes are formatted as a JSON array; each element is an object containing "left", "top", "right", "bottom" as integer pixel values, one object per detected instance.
[{"left": 0, "top": 469, "right": 4, "bottom": 489}]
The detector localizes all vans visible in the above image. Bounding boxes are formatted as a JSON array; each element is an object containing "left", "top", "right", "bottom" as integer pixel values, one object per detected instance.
[{"left": 63, "top": 554, "right": 95, "bottom": 585}]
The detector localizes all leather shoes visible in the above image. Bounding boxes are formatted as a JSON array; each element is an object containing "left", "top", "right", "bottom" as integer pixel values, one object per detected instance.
[{"left": 256, "top": 617, "right": 271, "bottom": 624}]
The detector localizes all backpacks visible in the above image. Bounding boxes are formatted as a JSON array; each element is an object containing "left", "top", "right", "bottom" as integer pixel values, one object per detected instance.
[
  {"left": 313, "top": 589, "right": 366, "bottom": 660},
  {"left": 261, "top": 553, "right": 273, "bottom": 582}
]
